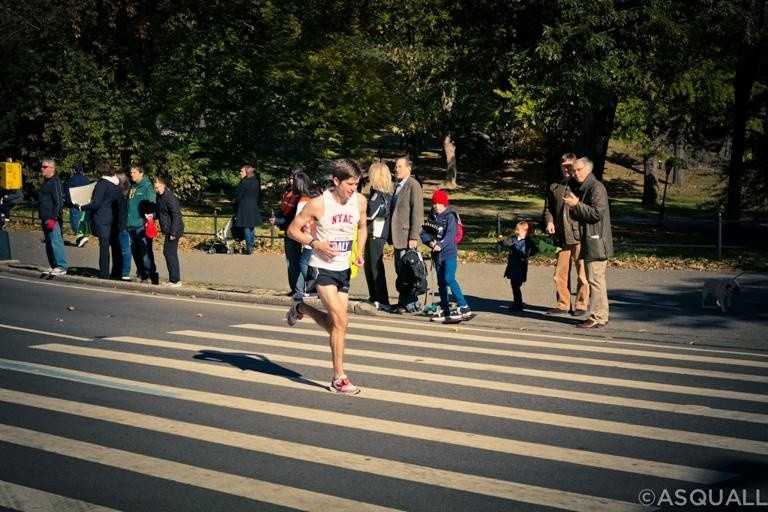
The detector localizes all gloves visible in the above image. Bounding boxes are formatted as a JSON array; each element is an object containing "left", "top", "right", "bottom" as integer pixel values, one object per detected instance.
[{"left": 45, "top": 218, "right": 55, "bottom": 230}]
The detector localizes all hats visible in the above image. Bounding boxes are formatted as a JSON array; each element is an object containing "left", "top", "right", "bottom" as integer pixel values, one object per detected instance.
[{"left": 431, "top": 191, "right": 448, "bottom": 206}]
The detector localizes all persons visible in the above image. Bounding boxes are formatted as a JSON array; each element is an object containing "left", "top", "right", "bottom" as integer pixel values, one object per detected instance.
[
  {"left": 563, "top": 156, "right": 613, "bottom": 329},
  {"left": 284, "top": 157, "right": 371, "bottom": 394},
  {"left": 230, "top": 164, "right": 264, "bottom": 255},
  {"left": 269, "top": 155, "right": 474, "bottom": 324},
  {"left": 0, "top": 158, "right": 183, "bottom": 284},
  {"left": 497, "top": 221, "right": 538, "bottom": 312},
  {"left": 542, "top": 153, "right": 591, "bottom": 315}
]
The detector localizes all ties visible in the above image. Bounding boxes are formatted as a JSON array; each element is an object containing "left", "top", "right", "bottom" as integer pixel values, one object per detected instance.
[{"left": 392, "top": 184, "right": 401, "bottom": 198}]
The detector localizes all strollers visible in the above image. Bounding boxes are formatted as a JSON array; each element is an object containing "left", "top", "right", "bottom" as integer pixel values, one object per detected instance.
[{"left": 209, "top": 214, "right": 244, "bottom": 255}]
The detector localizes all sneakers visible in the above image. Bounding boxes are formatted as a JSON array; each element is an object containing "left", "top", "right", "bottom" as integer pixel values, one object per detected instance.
[
  {"left": 329, "top": 374, "right": 360, "bottom": 394},
  {"left": 450, "top": 305, "right": 472, "bottom": 319},
  {"left": 287, "top": 300, "right": 303, "bottom": 326},
  {"left": 49, "top": 266, "right": 67, "bottom": 276},
  {"left": 373, "top": 301, "right": 392, "bottom": 311},
  {"left": 78, "top": 237, "right": 88, "bottom": 247},
  {"left": 432, "top": 307, "right": 453, "bottom": 320},
  {"left": 42, "top": 267, "right": 52, "bottom": 274},
  {"left": 162, "top": 281, "right": 181, "bottom": 288}
]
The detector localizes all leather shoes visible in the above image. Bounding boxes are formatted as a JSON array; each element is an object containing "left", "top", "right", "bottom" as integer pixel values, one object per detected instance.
[
  {"left": 548, "top": 308, "right": 568, "bottom": 314},
  {"left": 397, "top": 304, "right": 422, "bottom": 314},
  {"left": 577, "top": 319, "right": 604, "bottom": 328},
  {"left": 573, "top": 309, "right": 585, "bottom": 316}
]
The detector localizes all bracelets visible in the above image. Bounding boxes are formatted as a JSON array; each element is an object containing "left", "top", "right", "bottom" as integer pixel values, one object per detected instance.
[{"left": 308, "top": 236, "right": 320, "bottom": 247}]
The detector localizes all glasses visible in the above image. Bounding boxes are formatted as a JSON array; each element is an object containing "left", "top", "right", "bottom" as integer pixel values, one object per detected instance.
[{"left": 41, "top": 166, "right": 47, "bottom": 169}]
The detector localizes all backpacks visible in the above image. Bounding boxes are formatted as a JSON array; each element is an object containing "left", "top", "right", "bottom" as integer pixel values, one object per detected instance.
[{"left": 396, "top": 247, "right": 427, "bottom": 305}]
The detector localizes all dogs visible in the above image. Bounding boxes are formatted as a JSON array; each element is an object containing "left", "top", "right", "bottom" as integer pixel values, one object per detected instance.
[{"left": 702, "top": 278, "right": 742, "bottom": 313}]
